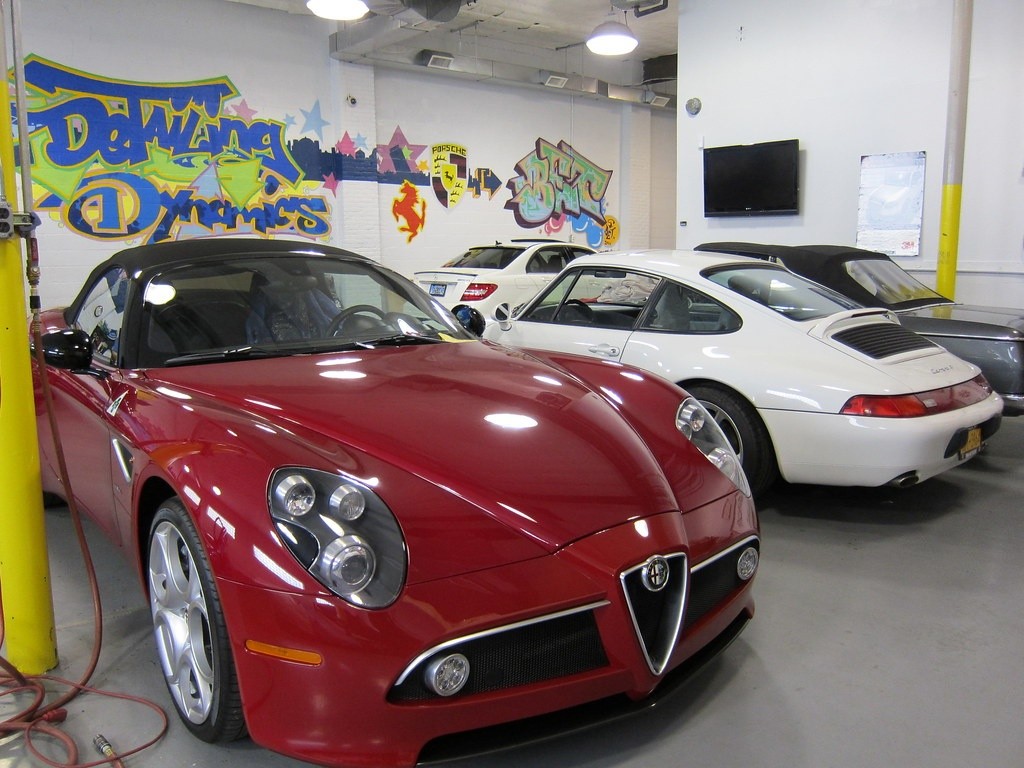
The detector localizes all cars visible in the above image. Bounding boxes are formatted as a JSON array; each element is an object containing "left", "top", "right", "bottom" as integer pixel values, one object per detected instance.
[{"left": 411, "top": 238, "right": 647, "bottom": 320}]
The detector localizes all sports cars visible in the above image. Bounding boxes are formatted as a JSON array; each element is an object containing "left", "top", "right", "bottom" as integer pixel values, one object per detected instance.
[
  {"left": 24, "top": 237, "right": 761, "bottom": 768},
  {"left": 578, "top": 241, "right": 1023, "bottom": 418},
  {"left": 421, "top": 249, "right": 1006, "bottom": 501}
]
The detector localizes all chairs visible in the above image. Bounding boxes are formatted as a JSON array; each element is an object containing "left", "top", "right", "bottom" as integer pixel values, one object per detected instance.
[
  {"left": 650, "top": 287, "right": 770, "bottom": 333},
  {"left": 152, "top": 266, "right": 336, "bottom": 357},
  {"left": 548, "top": 255, "right": 563, "bottom": 273}
]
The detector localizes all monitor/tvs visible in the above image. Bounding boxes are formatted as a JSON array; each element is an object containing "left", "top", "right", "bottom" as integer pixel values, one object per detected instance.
[{"left": 702, "top": 140, "right": 801, "bottom": 217}]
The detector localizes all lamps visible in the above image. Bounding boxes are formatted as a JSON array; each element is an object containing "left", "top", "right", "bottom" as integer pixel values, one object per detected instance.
[
  {"left": 306, "top": 0, "right": 371, "bottom": 20},
  {"left": 586, "top": 0, "right": 668, "bottom": 57}
]
